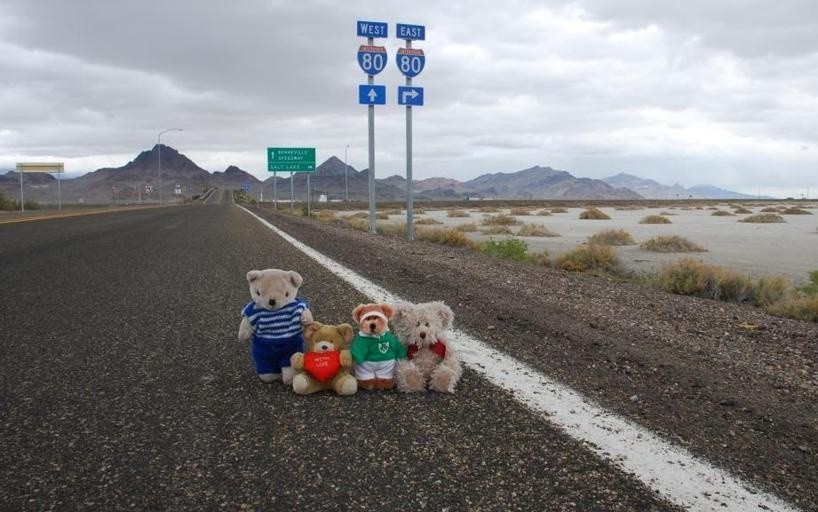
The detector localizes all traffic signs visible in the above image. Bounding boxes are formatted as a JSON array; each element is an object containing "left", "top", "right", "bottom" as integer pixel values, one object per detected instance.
[
  {"left": 357, "top": 45, "right": 387, "bottom": 75},
  {"left": 396, "top": 48, "right": 425, "bottom": 76},
  {"left": 268, "top": 148, "right": 315, "bottom": 171}
]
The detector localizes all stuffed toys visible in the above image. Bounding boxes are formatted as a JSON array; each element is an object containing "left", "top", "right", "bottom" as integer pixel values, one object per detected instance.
[
  {"left": 351, "top": 302, "right": 407, "bottom": 395},
  {"left": 392, "top": 299, "right": 465, "bottom": 397},
  {"left": 237, "top": 268, "right": 314, "bottom": 385},
  {"left": 288, "top": 318, "right": 361, "bottom": 400}
]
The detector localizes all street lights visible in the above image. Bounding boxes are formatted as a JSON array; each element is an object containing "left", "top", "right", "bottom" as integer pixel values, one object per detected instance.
[{"left": 158, "top": 129, "right": 184, "bottom": 205}]
